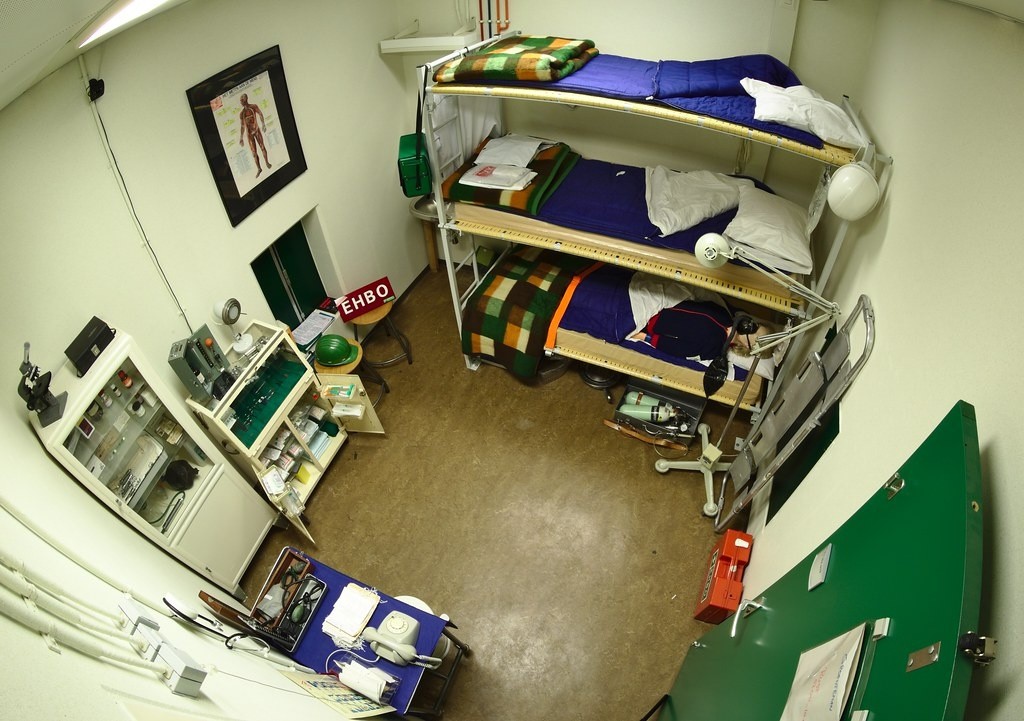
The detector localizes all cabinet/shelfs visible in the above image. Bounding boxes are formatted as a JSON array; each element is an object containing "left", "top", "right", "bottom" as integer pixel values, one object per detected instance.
[
  {"left": 28, "top": 322, "right": 290, "bottom": 603},
  {"left": 185, "top": 319, "right": 385, "bottom": 545}
]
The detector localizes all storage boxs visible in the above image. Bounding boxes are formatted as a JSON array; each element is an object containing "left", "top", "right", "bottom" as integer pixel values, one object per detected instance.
[
  {"left": 64, "top": 317, "right": 117, "bottom": 377},
  {"left": 397, "top": 132, "right": 431, "bottom": 198},
  {"left": 693, "top": 529, "right": 753, "bottom": 623}
]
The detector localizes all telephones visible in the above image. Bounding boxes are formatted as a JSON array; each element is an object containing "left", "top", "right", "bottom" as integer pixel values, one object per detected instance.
[{"left": 361, "top": 610, "right": 421, "bottom": 669}]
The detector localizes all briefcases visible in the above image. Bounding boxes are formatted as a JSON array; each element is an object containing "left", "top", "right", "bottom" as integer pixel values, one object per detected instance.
[{"left": 694, "top": 529, "right": 753, "bottom": 625}]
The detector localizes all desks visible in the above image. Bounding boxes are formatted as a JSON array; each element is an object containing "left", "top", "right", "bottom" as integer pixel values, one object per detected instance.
[{"left": 248, "top": 546, "right": 470, "bottom": 717}]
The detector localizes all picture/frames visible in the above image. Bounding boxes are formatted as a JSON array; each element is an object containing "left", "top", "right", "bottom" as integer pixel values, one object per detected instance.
[{"left": 186, "top": 44, "right": 308, "bottom": 227}]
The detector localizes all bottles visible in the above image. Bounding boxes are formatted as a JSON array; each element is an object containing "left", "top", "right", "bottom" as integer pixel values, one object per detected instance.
[
  {"left": 135, "top": 394, "right": 144, "bottom": 404},
  {"left": 98, "top": 390, "right": 113, "bottom": 407},
  {"left": 111, "top": 384, "right": 122, "bottom": 397},
  {"left": 119, "top": 370, "right": 133, "bottom": 389},
  {"left": 127, "top": 401, "right": 146, "bottom": 418},
  {"left": 190, "top": 367, "right": 207, "bottom": 389}
]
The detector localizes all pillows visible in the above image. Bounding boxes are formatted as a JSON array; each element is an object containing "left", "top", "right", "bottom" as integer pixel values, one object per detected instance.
[
  {"left": 784, "top": 85, "right": 865, "bottom": 150},
  {"left": 728, "top": 309, "right": 786, "bottom": 378},
  {"left": 740, "top": 76, "right": 816, "bottom": 137},
  {"left": 722, "top": 183, "right": 814, "bottom": 275}
]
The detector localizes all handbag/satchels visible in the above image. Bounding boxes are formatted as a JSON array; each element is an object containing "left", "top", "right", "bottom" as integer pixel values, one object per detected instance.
[{"left": 398, "top": 64, "right": 433, "bottom": 197}]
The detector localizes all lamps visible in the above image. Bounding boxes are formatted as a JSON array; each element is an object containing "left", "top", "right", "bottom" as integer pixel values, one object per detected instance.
[
  {"left": 211, "top": 298, "right": 254, "bottom": 353},
  {"left": 826, "top": 144, "right": 893, "bottom": 223},
  {"left": 654, "top": 233, "right": 840, "bottom": 519}
]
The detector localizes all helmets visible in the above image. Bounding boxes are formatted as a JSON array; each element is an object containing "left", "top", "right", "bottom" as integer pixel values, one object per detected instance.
[
  {"left": 315, "top": 334, "right": 358, "bottom": 366},
  {"left": 827, "top": 164, "right": 880, "bottom": 221}
]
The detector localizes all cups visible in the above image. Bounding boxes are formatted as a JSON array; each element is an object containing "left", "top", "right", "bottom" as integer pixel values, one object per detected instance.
[{"left": 86, "top": 399, "right": 103, "bottom": 421}]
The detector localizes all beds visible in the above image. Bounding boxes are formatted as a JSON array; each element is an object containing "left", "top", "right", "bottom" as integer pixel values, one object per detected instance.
[{"left": 415, "top": 38, "right": 876, "bottom": 429}]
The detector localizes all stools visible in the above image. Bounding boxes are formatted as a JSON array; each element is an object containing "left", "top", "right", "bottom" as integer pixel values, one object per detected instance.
[
  {"left": 313, "top": 338, "right": 389, "bottom": 409},
  {"left": 350, "top": 300, "right": 412, "bottom": 366},
  {"left": 393, "top": 595, "right": 451, "bottom": 662}
]
